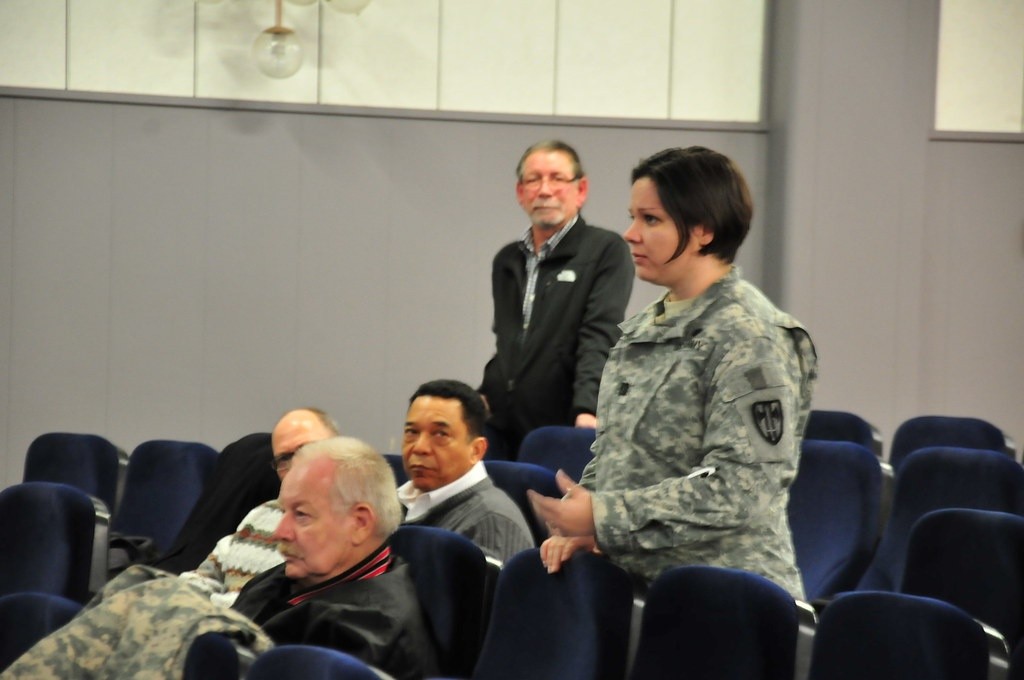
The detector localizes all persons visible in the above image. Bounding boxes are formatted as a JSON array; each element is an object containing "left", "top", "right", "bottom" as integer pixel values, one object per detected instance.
[
  {"left": 396, "top": 379, "right": 535, "bottom": 572},
  {"left": 475, "top": 144, "right": 635, "bottom": 462},
  {"left": 183, "top": 408, "right": 348, "bottom": 610},
  {"left": 230, "top": 437, "right": 442, "bottom": 680},
  {"left": 527, "top": 145, "right": 819, "bottom": 605}
]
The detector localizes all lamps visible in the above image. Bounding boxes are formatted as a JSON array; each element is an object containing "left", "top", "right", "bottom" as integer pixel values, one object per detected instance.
[{"left": 253, "top": 0, "right": 369, "bottom": 80}]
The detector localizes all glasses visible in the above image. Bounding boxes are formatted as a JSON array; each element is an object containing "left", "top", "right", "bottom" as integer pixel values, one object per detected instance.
[
  {"left": 520, "top": 174, "right": 577, "bottom": 193},
  {"left": 271, "top": 457, "right": 291, "bottom": 472}
]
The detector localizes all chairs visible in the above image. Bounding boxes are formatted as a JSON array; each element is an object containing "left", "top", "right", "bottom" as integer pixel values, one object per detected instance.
[{"left": 0, "top": 408, "right": 1024, "bottom": 679}]
